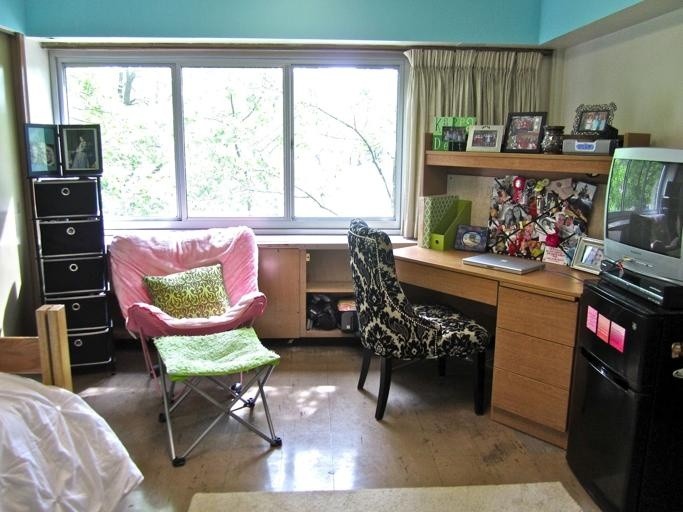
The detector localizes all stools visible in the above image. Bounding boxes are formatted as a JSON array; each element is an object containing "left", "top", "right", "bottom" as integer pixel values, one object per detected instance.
[{"left": 151, "top": 325, "right": 282, "bottom": 467}]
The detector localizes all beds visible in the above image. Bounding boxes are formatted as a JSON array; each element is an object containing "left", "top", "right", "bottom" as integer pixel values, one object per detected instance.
[{"left": 0, "top": 304, "right": 73, "bottom": 512}]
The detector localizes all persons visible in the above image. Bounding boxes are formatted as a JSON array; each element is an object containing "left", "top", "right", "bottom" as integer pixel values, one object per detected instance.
[
  {"left": 585, "top": 115, "right": 593, "bottom": 129},
  {"left": 73, "top": 135, "right": 90, "bottom": 168},
  {"left": 592, "top": 113, "right": 599, "bottom": 130},
  {"left": 587, "top": 248, "right": 603, "bottom": 266},
  {"left": 500, "top": 206, "right": 575, "bottom": 239}
]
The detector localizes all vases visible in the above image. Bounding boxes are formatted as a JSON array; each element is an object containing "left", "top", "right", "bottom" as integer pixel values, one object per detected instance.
[{"left": 542, "top": 124, "right": 565, "bottom": 153}]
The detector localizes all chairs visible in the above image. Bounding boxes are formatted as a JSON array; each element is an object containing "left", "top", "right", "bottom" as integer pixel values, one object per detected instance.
[
  {"left": 109, "top": 226, "right": 268, "bottom": 405},
  {"left": 347, "top": 217, "right": 491, "bottom": 420}
]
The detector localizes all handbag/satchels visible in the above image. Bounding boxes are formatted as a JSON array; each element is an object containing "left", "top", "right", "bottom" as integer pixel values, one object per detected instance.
[
  {"left": 308, "top": 294, "right": 336, "bottom": 330},
  {"left": 336, "top": 311, "right": 358, "bottom": 333}
]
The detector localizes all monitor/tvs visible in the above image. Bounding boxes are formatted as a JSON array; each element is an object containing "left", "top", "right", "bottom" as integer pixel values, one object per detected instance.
[{"left": 604, "top": 146, "right": 683, "bottom": 287}]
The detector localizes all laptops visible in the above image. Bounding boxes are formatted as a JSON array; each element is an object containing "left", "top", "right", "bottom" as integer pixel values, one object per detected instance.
[{"left": 463, "top": 252, "right": 545, "bottom": 275}]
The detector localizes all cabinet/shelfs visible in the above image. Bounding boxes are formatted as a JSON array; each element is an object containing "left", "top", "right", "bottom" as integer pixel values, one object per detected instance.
[
  {"left": 566, "top": 277, "right": 683, "bottom": 512},
  {"left": 109, "top": 234, "right": 417, "bottom": 343},
  {"left": 392, "top": 133, "right": 652, "bottom": 450},
  {"left": 30, "top": 175, "right": 115, "bottom": 373}
]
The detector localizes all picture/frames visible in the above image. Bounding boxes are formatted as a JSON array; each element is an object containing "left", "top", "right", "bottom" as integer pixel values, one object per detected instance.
[
  {"left": 502, "top": 112, "right": 547, "bottom": 153},
  {"left": 466, "top": 123, "right": 505, "bottom": 154},
  {"left": 572, "top": 102, "right": 617, "bottom": 135},
  {"left": 60, "top": 122, "right": 103, "bottom": 177},
  {"left": 571, "top": 235, "right": 605, "bottom": 276},
  {"left": 23, "top": 123, "right": 61, "bottom": 178}
]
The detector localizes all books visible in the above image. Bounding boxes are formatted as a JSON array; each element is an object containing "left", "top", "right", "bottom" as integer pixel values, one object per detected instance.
[{"left": 417, "top": 194, "right": 458, "bottom": 248}]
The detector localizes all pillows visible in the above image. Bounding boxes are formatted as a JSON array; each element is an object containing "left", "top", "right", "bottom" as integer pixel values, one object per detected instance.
[{"left": 142, "top": 263, "right": 231, "bottom": 319}]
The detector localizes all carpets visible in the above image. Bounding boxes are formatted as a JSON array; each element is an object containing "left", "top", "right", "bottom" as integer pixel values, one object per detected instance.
[{"left": 186, "top": 481, "right": 584, "bottom": 511}]
